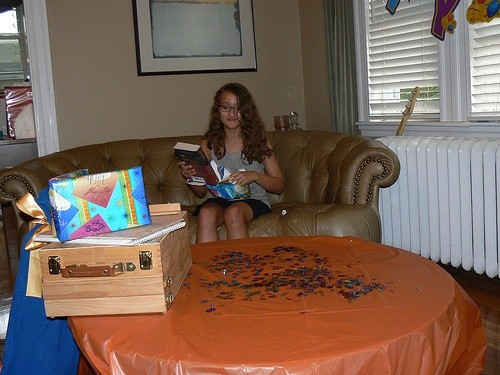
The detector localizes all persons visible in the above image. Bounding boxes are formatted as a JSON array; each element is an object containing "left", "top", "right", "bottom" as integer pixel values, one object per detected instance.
[{"left": 179, "top": 83, "right": 285, "bottom": 243}]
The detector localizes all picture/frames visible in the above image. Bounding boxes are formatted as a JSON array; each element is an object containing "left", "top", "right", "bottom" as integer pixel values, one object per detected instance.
[{"left": 132, "top": 0, "right": 257, "bottom": 76}]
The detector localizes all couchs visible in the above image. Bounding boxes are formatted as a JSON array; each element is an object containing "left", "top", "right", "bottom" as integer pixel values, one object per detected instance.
[{"left": 0, "top": 129, "right": 401, "bottom": 284}]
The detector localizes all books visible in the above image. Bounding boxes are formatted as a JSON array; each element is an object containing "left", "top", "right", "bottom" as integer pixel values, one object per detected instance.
[
  {"left": 174, "top": 142, "right": 219, "bottom": 186},
  {"left": 33, "top": 221, "right": 186, "bottom": 246}
]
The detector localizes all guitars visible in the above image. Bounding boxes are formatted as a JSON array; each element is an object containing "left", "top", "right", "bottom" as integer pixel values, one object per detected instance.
[{"left": 396, "top": 86, "right": 420, "bottom": 136}]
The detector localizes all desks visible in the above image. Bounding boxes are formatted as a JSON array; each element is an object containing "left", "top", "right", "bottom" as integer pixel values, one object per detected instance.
[{"left": 67, "top": 236, "right": 488, "bottom": 375}]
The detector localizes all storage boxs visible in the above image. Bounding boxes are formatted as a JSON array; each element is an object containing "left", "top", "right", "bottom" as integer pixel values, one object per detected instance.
[{"left": 38, "top": 211, "right": 193, "bottom": 319}]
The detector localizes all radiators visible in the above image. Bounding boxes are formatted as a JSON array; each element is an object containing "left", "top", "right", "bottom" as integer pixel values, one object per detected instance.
[{"left": 375, "top": 135, "right": 500, "bottom": 279}]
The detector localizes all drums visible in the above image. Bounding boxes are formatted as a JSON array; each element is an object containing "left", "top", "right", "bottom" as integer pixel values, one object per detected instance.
[{"left": 274, "top": 115, "right": 290, "bottom": 132}]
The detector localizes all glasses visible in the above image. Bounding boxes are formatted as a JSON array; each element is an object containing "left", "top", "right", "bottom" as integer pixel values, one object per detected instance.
[{"left": 218, "top": 104, "right": 240, "bottom": 113}]
